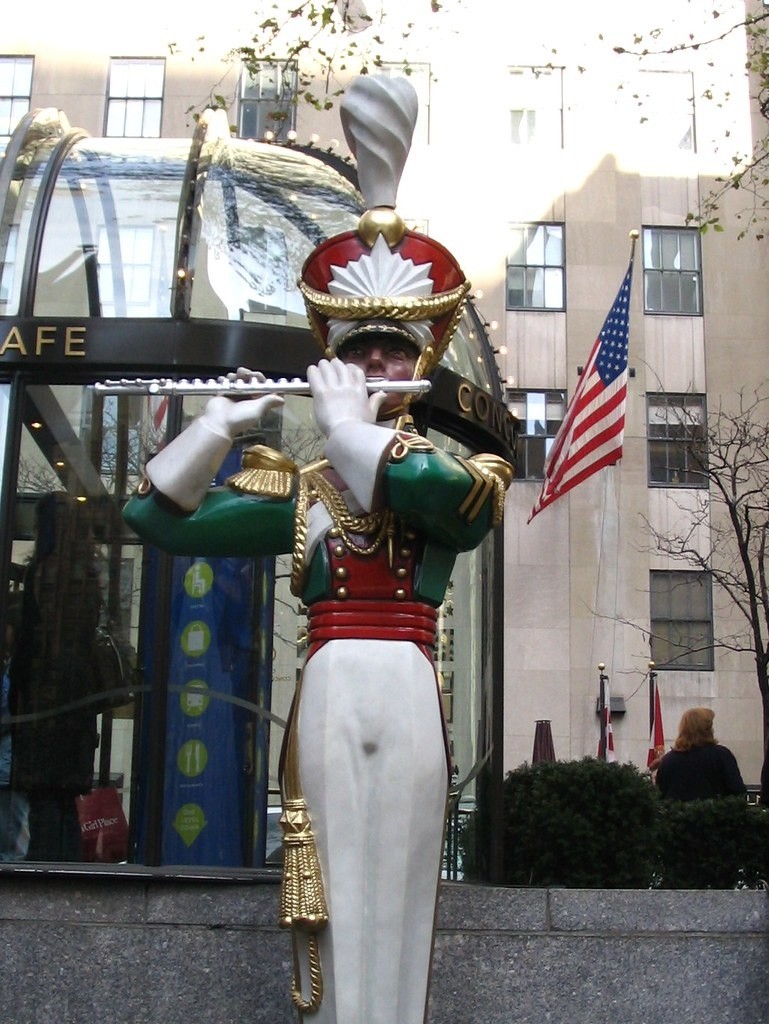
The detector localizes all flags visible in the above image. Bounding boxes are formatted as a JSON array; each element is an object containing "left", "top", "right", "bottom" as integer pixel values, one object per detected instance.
[
  {"left": 647, "top": 676, "right": 665, "bottom": 785},
  {"left": 527, "top": 258, "right": 633, "bottom": 525},
  {"left": 598, "top": 676, "right": 616, "bottom": 764}
]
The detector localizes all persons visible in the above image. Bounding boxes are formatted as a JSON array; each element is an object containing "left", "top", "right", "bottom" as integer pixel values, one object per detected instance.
[
  {"left": 0, "top": 492, "right": 128, "bottom": 863},
  {"left": 656, "top": 707, "right": 747, "bottom": 802},
  {"left": 120, "top": 212, "right": 513, "bottom": 1024}
]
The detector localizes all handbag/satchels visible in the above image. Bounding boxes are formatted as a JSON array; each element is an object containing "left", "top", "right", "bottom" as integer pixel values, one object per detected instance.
[
  {"left": 74, "top": 784, "right": 128, "bottom": 864},
  {"left": 89, "top": 627, "right": 131, "bottom": 714}
]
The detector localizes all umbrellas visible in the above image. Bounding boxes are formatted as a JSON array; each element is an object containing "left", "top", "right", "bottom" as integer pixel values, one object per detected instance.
[{"left": 532, "top": 719, "right": 556, "bottom": 763}]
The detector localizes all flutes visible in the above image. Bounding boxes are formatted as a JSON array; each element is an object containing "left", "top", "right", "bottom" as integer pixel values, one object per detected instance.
[{"left": 93, "top": 375, "right": 432, "bottom": 396}]
